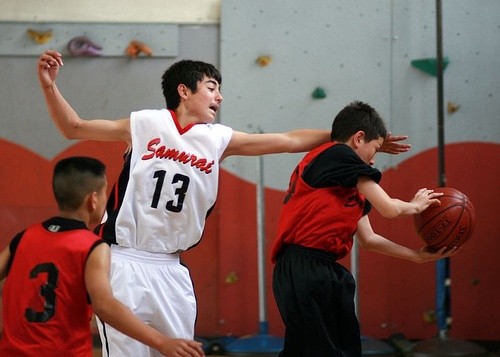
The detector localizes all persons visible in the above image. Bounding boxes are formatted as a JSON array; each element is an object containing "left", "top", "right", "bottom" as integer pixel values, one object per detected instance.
[
  {"left": 35, "top": 48, "right": 412, "bottom": 357},
  {"left": 1, "top": 155, "right": 207, "bottom": 357},
  {"left": 267, "top": 100, "right": 465, "bottom": 357}
]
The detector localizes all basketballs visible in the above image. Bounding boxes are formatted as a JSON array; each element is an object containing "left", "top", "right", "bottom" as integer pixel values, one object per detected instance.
[{"left": 413, "top": 187, "right": 476, "bottom": 251}]
